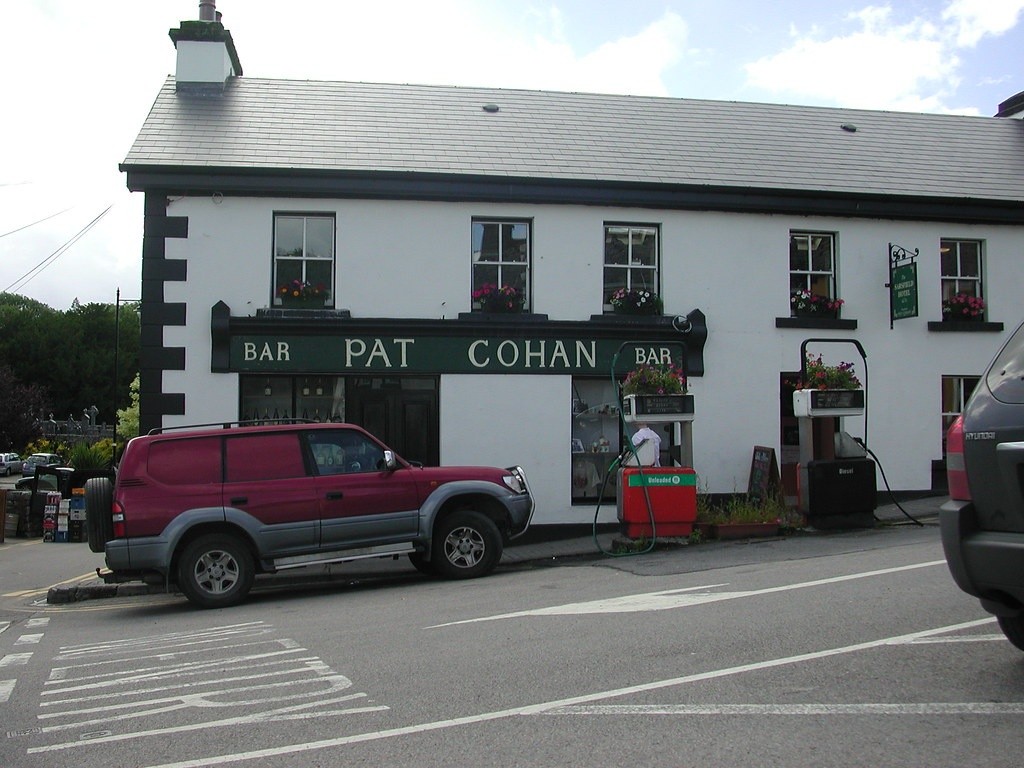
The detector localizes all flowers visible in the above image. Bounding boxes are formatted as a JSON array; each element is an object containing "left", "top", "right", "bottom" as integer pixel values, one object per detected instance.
[
  {"left": 791, "top": 288, "right": 845, "bottom": 314},
  {"left": 941, "top": 291, "right": 986, "bottom": 316},
  {"left": 783, "top": 349, "right": 863, "bottom": 392},
  {"left": 609, "top": 287, "right": 663, "bottom": 309},
  {"left": 472, "top": 281, "right": 526, "bottom": 310},
  {"left": 618, "top": 360, "right": 692, "bottom": 396},
  {"left": 280, "top": 280, "right": 331, "bottom": 303}
]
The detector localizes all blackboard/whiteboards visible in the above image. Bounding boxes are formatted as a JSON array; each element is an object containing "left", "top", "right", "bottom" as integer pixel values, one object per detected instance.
[{"left": 747, "top": 446, "right": 774, "bottom": 498}]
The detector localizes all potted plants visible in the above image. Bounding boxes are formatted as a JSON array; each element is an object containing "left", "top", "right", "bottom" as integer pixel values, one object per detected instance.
[
  {"left": 61, "top": 441, "right": 112, "bottom": 488},
  {"left": 692, "top": 472, "right": 805, "bottom": 541}
]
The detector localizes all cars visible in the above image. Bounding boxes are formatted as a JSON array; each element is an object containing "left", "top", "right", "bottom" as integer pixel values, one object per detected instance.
[
  {"left": 15, "top": 463, "right": 67, "bottom": 491},
  {"left": 21, "top": 452, "right": 65, "bottom": 476},
  {"left": 0, "top": 451, "right": 29, "bottom": 477},
  {"left": 939, "top": 316, "right": 1024, "bottom": 660}
]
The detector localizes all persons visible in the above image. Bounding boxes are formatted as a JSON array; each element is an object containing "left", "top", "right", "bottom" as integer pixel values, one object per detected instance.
[{"left": 631, "top": 423, "right": 661, "bottom": 467}]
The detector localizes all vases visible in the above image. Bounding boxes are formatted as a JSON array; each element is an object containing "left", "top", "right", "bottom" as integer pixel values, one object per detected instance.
[
  {"left": 614, "top": 305, "right": 658, "bottom": 316},
  {"left": 480, "top": 302, "right": 524, "bottom": 312},
  {"left": 281, "top": 298, "right": 325, "bottom": 309},
  {"left": 793, "top": 387, "right": 867, "bottom": 419},
  {"left": 795, "top": 311, "right": 838, "bottom": 318},
  {"left": 943, "top": 314, "right": 984, "bottom": 322},
  {"left": 622, "top": 393, "right": 695, "bottom": 426}
]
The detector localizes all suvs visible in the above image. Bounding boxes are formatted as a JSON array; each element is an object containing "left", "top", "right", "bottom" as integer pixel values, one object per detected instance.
[{"left": 85, "top": 417, "right": 535, "bottom": 608}]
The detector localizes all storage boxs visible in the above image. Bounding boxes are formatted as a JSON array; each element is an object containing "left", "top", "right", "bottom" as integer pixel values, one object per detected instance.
[{"left": 43, "top": 487, "right": 88, "bottom": 543}]
